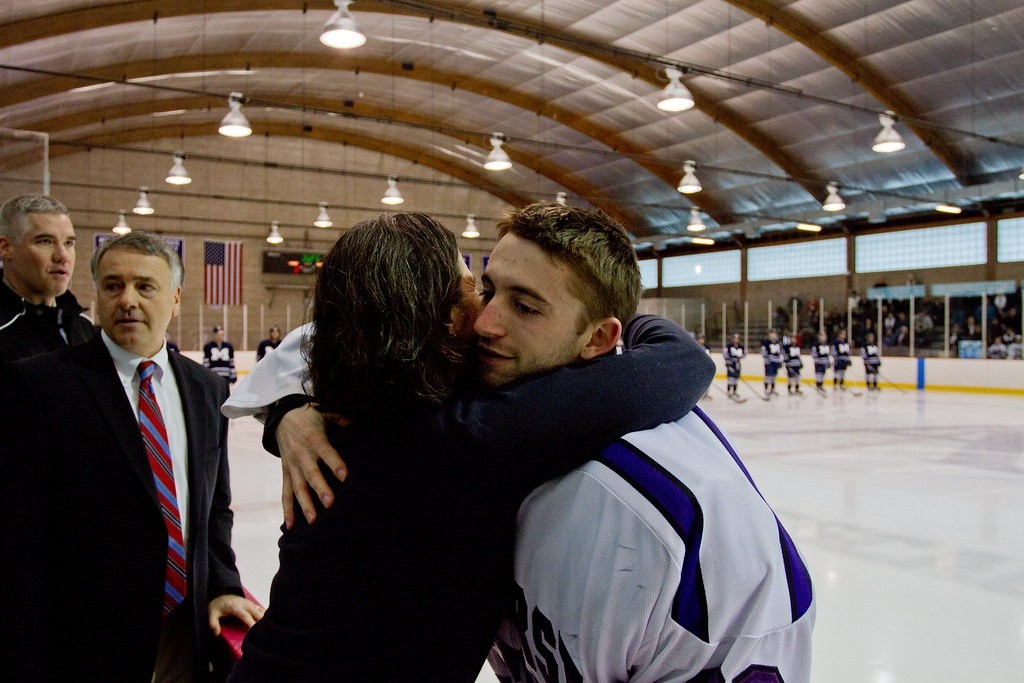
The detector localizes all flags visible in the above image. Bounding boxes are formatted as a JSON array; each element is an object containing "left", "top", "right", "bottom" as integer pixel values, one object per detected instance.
[{"left": 204, "top": 241, "right": 244, "bottom": 305}]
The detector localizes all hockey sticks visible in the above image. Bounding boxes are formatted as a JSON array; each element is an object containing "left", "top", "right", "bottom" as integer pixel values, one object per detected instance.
[
  {"left": 712, "top": 381, "right": 748, "bottom": 404},
  {"left": 780, "top": 366, "right": 805, "bottom": 397},
  {"left": 866, "top": 364, "right": 907, "bottom": 394},
  {"left": 728, "top": 366, "right": 769, "bottom": 401},
  {"left": 830, "top": 367, "right": 862, "bottom": 397},
  {"left": 788, "top": 366, "right": 828, "bottom": 398}
]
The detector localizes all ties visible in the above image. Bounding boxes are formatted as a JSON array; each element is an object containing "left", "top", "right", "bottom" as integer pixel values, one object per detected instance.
[{"left": 139, "top": 360, "right": 187, "bottom": 617}]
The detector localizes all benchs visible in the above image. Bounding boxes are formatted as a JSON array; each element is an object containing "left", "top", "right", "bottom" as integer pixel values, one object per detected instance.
[{"left": 705, "top": 320, "right": 786, "bottom": 354}]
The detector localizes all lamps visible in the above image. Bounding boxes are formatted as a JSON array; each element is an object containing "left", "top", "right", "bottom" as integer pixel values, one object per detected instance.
[
  {"left": 132, "top": 186, "right": 155, "bottom": 215},
  {"left": 164, "top": 151, "right": 192, "bottom": 185},
  {"left": 267, "top": 221, "right": 284, "bottom": 244},
  {"left": 677, "top": 159, "right": 703, "bottom": 194},
  {"left": 872, "top": 108, "right": 905, "bottom": 153},
  {"left": 380, "top": 176, "right": 405, "bottom": 205},
  {"left": 555, "top": 191, "right": 567, "bottom": 206},
  {"left": 483, "top": 131, "right": 512, "bottom": 171},
  {"left": 217, "top": 92, "right": 253, "bottom": 138},
  {"left": 822, "top": 181, "right": 846, "bottom": 212},
  {"left": 313, "top": 201, "right": 332, "bottom": 228},
  {"left": 687, "top": 207, "right": 705, "bottom": 232},
  {"left": 461, "top": 214, "right": 480, "bottom": 239},
  {"left": 320, "top": 0, "right": 367, "bottom": 49},
  {"left": 656, "top": 67, "right": 694, "bottom": 112},
  {"left": 112, "top": 209, "right": 132, "bottom": 235}
]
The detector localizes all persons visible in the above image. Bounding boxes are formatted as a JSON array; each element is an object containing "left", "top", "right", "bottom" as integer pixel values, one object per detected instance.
[
  {"left": 221, "top": 203, "right": 816, "bottom": 683},
  {"left": 812, "top": 332, "right": 831, "bottom": 390},
  {"left": 861, "top": 334, "right": 881, "bottom": 390},
  {"left": 832, "top": 330, "right": 851, "bottom": 391},
  {"left": 0, "top": 192, "right": 265, "bottom": 683},
  {"left": 698, "top": 335, "right": 711, "bottom": 356},
  {"left": 203, "top": 326, "right": 237, "bottom": 384},
  {"left": 762, "top": 329, "right": 804, "bottom": 395},
  {"left": 723, "top": 333, "right": 746, "bottom": 397},
  {"left": 226, "top": 209, "right": 716, "bottom": 683},
  {"left": 257, "top": 327, "right": 281, "bottom": 362},
  {"left": 797, "top": 290, "right": 1024, "bottom": 359}
]
